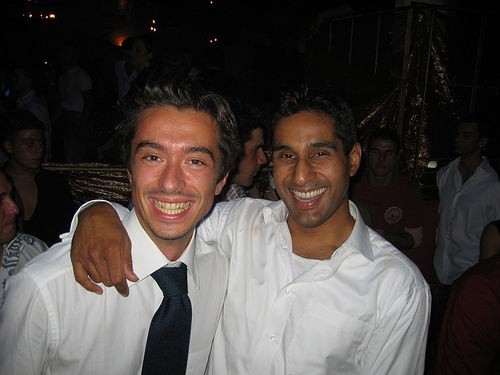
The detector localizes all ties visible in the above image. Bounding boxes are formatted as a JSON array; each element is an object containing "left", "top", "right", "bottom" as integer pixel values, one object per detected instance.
[{"left": 141, "top": 263, "right": 192, "bottom": 375}]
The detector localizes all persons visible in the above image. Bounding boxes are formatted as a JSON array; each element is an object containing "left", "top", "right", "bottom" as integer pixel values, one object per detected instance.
[
  {"left": 349, "top": 128, "right": 424, "bottom": 250},
  {"left": 423, "top": 114, "right": 500, "bottom": 375},
  {"left": 0, "top": 82, "right": 229, "bottom": 375},
  {"left": 210, "top": 106, "right": 267, "bottom": 204},
  {"left": 59, "top": 87, "right": 432, "bottom": 375},
  {"left": 0, "top": 167, "right": 49, "bottom": 308},
  {"left": 0, "top": 105, "right": 76, "bottom": 248}
]
